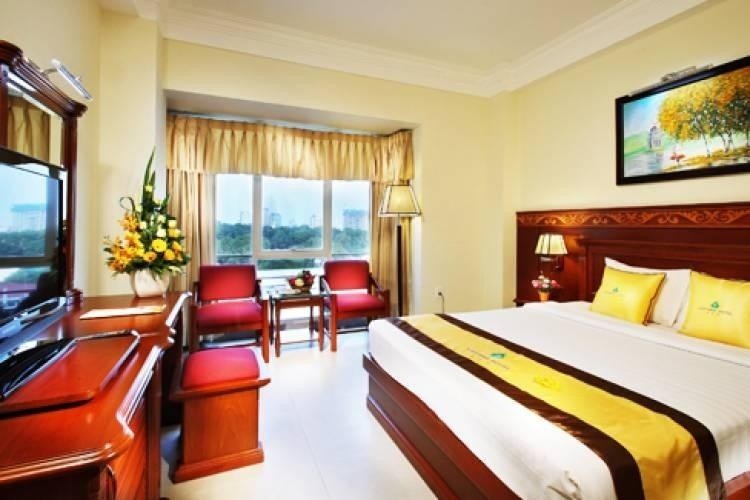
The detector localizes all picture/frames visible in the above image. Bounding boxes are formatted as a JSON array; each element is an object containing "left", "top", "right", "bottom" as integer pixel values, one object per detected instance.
[{"left": 615, "top": 56, "right": 750, "bottom": 184}]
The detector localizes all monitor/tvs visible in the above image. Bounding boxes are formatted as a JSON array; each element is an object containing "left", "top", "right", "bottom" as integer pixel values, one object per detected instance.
[{"left": 0, "top": 147, "right": 78, "bottom": 398}]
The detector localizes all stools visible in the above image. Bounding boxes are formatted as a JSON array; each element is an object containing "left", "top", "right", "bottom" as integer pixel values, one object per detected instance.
[{"left": 167, "top": 347, "right": 271, "bottom": 483}]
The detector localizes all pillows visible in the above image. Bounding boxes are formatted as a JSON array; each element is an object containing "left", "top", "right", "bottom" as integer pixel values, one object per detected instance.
[
  {"left": 590, "top": 266, "right": 666, "bottom": 327},
  {"left": 605, "top": 257, "right": 692, "bottom": 327},
  {"left": 672, "top": 288, "right": 688, "bottom": 330},
  {"left": 678, "top": 272, "right": 750, "bottom": 349}
]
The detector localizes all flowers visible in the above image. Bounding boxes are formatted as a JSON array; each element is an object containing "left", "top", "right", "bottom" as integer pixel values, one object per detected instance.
[
  {"left": 288, "top": 270, "right": 315, "bottom": 287},
  {"left": 531, "top": 275, "right": 561, "bottom": 292},
  {"left": 100, "top": 144, "right": 192, "bottom": 282}
]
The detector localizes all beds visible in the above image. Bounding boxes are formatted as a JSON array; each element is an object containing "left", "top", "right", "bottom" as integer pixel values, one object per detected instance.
[{"left": 362, "top": 293, "right": 750, "bottom": 500}]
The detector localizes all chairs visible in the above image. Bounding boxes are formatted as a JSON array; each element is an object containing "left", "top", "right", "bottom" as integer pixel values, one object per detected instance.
[
  {"left": 320, "top": 260, "right": 390, "bottom": 351},
  {"left": 189, "top": 265, "right": 269, "bottom": 363}
]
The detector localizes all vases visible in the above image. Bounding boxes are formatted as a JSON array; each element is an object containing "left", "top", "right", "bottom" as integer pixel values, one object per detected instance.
[
  {"left": 134, "top": 269, "right": 170, "bottom": 298},
  {"left": 538, "top": 290, "right": 549, "bottom": 303},
  {"left": 299, "top": 286, "right": 310, "bottom": 292}
]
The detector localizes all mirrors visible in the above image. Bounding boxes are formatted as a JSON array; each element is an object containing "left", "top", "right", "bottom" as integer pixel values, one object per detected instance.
[{"left": 0, "top": 39, "right": 87, "bottom": 299}]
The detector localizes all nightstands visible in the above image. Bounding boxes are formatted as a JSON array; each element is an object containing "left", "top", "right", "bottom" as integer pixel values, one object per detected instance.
[{"left": 513, "top": 296, "right": 558, "bottom": 307}]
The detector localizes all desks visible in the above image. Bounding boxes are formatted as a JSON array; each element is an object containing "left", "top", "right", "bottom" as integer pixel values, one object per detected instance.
[{"left": 0, "top": 289, "right": 191, "bottom": 485}]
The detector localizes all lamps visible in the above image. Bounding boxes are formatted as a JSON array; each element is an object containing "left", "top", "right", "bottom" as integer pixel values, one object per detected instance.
[
  {"left": 534, "top": 234, "right": 568, "bottom": 278},
  {"left": 378, "top": 184, "right": 421, "bottom": 317},
  {"left": 40, "top": 55, "right": 92, "bottom": 101}
]
